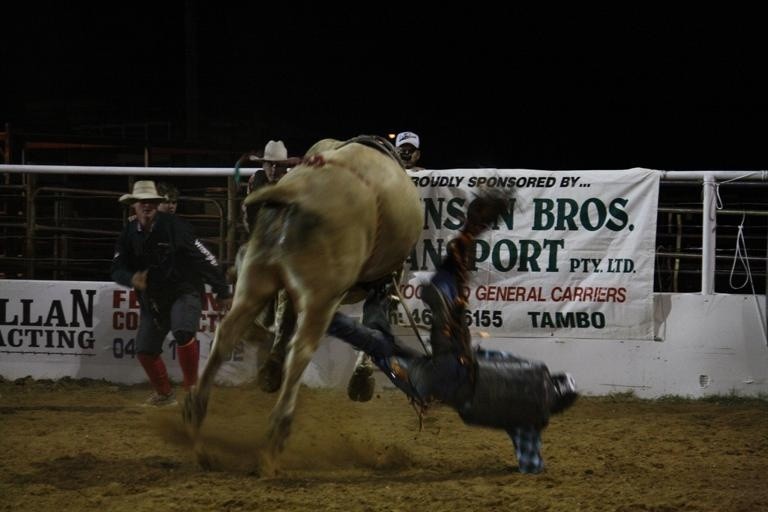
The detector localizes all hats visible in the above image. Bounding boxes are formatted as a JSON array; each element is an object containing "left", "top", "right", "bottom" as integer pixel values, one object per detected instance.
[
  {"left": 395, "top": 131, "right": 420, "bottom": 149},
  {"left": 555, "top": 370, "right": 580, "bottom": 414},
  {"left": 249, "top": 140, "right": 301, "bottom": 166},
  {"left": 118, "top": 180, "right": 169, "bottom": 204}
]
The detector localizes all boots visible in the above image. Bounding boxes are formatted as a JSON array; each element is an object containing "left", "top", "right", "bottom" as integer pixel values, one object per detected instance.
[
  {"left": 137, "top": 357, "right": 176, "bottom": 409},
  {"left": 173, "top": 335, "right": 200, "bottom": 396}
]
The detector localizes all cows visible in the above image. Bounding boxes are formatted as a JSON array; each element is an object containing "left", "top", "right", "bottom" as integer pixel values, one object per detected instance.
[{"left": 182, "top": 137, "right": 424, "bottom": 480}]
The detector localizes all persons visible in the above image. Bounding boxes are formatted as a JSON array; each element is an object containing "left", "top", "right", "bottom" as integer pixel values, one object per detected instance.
[
  {"left": 110, "top": 179, "right": 232, "bottom": 411},
  {"left": 158, "top": 184, "right": 180, "bottom": 213},
  {"left": 247, "top": 137, "right": 301, "bottom": 195},
  {"left": 324, "top": 182, "right": 579, "bottom": 476},
  {"left": 392, "top": 129, "right": 426, "bottom": 174}
]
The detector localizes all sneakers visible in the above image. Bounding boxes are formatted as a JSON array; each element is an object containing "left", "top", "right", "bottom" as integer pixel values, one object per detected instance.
[{"left": 420, "top": 282, "right": 452, "bottom": 335}]
